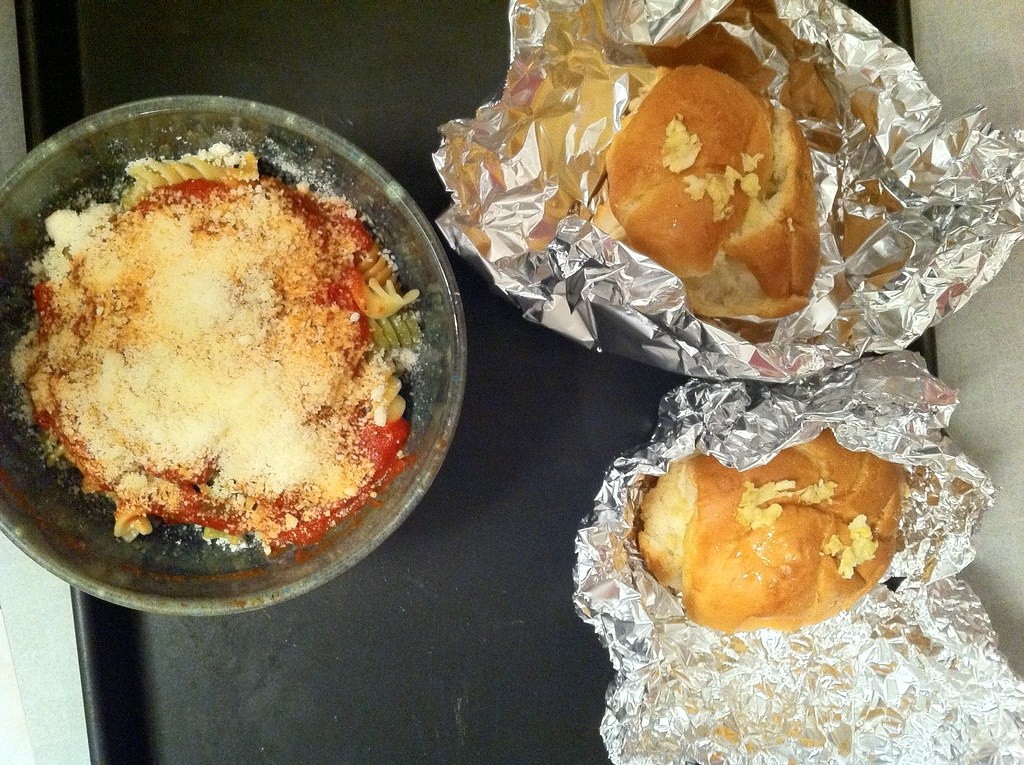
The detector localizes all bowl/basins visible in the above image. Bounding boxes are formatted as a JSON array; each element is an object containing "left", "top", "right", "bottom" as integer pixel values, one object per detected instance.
[{"left": 0, "top": 91, "right": 466, "bottom": 617}]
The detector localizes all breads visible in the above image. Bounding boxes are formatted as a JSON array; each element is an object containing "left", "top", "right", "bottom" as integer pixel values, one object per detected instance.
[
  {"left": 601, "top": 60, "right": 822, "bottom": 322},
  {"left": 640, "top": 427, "right": 904, "bottom": 630}
]
